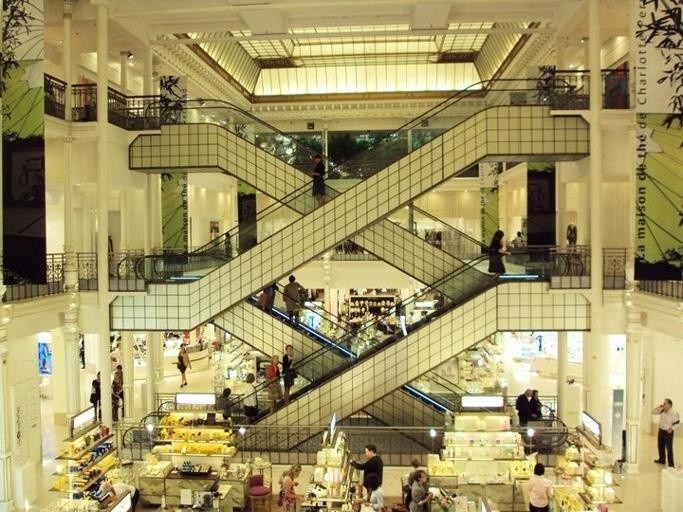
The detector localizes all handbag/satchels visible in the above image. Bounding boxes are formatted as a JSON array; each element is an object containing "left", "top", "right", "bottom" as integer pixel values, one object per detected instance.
[{"left": 278, "top": 491, "right": 282, "bottom": 506}]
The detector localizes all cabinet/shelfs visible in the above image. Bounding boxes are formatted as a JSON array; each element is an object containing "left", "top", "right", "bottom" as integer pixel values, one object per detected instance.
[
  {"left": 49, "top": 411, "right": 623, "bottom": 512},
  {"left": 217, "top": 332, "right": 275, "bottom": 398},
  {"left": 348, "top": 295, "right": 396, "bottom": 325},
  {"left": 464, "top": 335, "right": 506, "bottom": 395}
]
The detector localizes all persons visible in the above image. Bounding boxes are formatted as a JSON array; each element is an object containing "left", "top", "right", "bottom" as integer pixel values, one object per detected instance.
[
  {"left": 80, "top": 341, "right": 123, "bottom": 423},
  {"left": 651, "top": 398, "right": 680, "bottom": 471},
  {"left": 280, "top": 445, "right": 431, "bottom": 512},
  {"left": 335, "top": 239, "right": 364, "bottom": 255},
  {"left": 176, "top": 343, "right": 192, "bottom": 387},
  {"left": 529, "top": 462, "right": 554, "bottom": 511},
  {"left": 262, "top": 275, "right": 319, "bottom": 325},
  {"left": 515, "top": 231, "right": 525, "bottom": 249},
  {"left": 566, "top": 220, "right": 578, "bottom": 253},
  {"left": 488, "top": 229, "right": 511, "bottom": 283},
  {"left": 224, "top": 232, "right": 233, "bottom": 258},
  {"left": 99, "top": 483, "right": 140, "bottom": 511},
  {"left": 515, "top": 388, "right": 545, "bottom": 439},
  {"left": 309, "top": 154, "right": 325, "bottom": 206},
  {"left": 108, "top": 234, "right": 116, "bottom": 277},
  {"left": 220, "top": 343, "right": 295, "bottom": 424}
]
